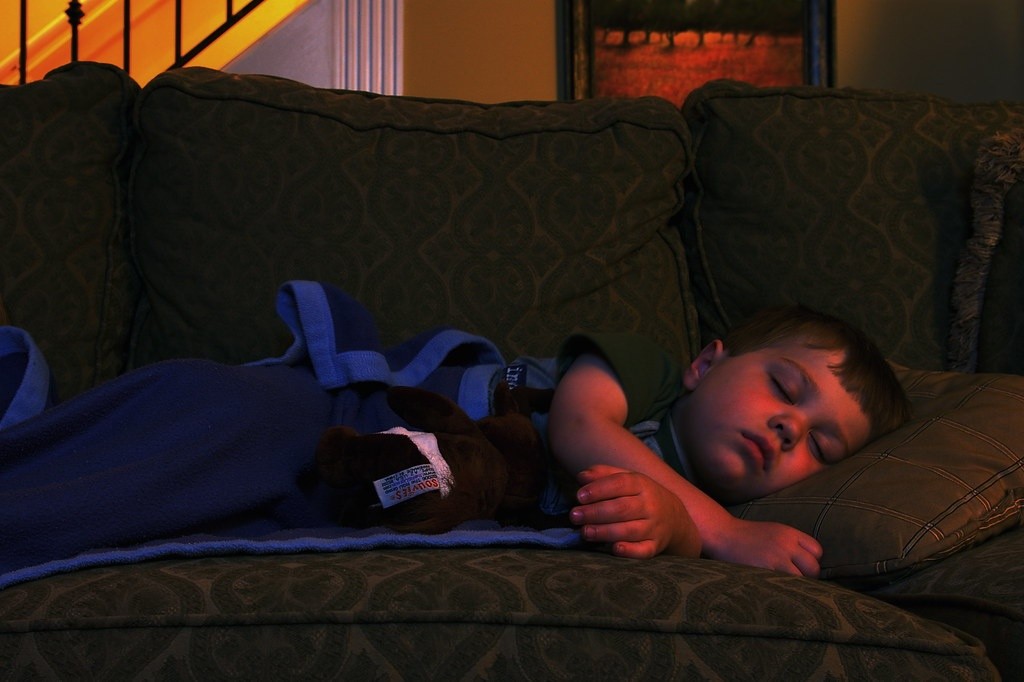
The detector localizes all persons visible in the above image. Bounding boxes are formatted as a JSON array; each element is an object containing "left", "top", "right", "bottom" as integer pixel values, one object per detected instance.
[{"left": 506, "top": 304, "right": 906, "bottom": 579}]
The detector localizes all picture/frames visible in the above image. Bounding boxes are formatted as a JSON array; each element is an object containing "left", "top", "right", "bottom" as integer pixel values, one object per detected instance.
[{"left": 554, "top": 0, "right": 837, "bottom": 105}]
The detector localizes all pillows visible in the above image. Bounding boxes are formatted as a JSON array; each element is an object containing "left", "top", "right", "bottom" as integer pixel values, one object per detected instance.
[
  {"left": 945, "top": 127, "right": 1024, "bottom": 378},
  {"left": 722, "top": 357, "right": 1024, "bottom": 588}
]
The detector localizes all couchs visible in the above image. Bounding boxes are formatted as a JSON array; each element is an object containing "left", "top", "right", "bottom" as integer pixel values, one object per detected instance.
[{"left": 1, "top": 62, "right": 1024, "bottom": 682}]
